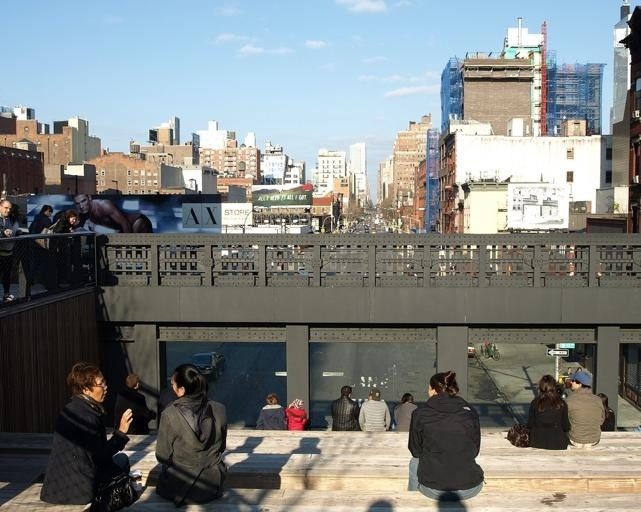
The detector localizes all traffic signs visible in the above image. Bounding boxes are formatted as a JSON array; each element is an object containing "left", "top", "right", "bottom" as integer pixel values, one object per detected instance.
[
  {"left": 560, "top": 343, "right": 576, "bottom": 349},
  {"left": 547, "top": 349, "right": 570, "bottom": 357}
]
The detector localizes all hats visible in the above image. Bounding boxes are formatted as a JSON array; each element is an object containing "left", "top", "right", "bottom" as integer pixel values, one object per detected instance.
[
  {"left": 569, "top": 372, "right": 592, "bottom": 387},
  {"left": 370, "top": 389, "right": 381, "bottom": 401}
]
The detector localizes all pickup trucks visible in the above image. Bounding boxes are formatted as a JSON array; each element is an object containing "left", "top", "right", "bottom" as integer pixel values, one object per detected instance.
[{"left": 192, "top": 351, "right": 227, "bottom": 379}]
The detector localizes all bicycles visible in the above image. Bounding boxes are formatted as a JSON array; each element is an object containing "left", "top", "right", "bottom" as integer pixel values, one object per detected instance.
[{"left": 484, "top": 343, "right": 501, "bottom": 360}]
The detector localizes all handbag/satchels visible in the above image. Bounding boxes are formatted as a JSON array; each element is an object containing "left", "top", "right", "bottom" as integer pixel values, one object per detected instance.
[
  {"left": 90, "top": 471, "right": 141, "bottom": 512},
  {"left": 507, "top": 422, "right": 530, "bottom": 447}
]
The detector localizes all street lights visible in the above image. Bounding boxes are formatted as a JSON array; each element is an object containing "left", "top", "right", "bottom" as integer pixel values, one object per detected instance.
[
  {"left": 400, "top": 215, "right": 422, "bottom": 234},
  {"left": 244, "top": 208, "right": 263, "bottom": 233},
  {"left": 320, "top": 212, "right": 354, "bottom": 233},
  {"left": 111, "top": 179, "right": 119, "bottom": 195},
  {"left": 189, "top": 178, "right": 198, "bottom": 193}
]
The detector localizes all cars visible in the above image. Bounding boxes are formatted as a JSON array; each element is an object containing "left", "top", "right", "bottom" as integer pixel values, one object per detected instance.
[
  {"left": 347, "top": 219, "right": 381, "bottom": 233},
  {"left": 468, "top": 343, "right": 476, "bottom": 358}
]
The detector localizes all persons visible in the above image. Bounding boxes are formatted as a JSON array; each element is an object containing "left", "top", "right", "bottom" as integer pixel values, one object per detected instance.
[
  {"left": 114, "top": 372, "right": 157, "bottom": 435},
  {"left": 53, "top": 193, "right": 154, "bottom": 234},
  {"left": 255, "top": 392, "right": 288, "bottom": 430},
  {"left": 563, "top": 367, "right": 574, "bottom": 376},
  {"left": 29, "top": 204, "right": 54, "bottom": 240},
  {"left": 565, "top": 369, "right": 606, "bottom": 449},
  {"left": 358, "top": 388, "right": 392, "bottom": 431},
  {"left": 40, "top": 358, "right": 134, "bottom": 505},
  {"left": 563, "top": 377, "right": 574, "bottom": 397},
  {"left": 597, "top": 393, "right": 615, "bottom": 431},
  {"left": 156, "top": 363, "right": 228, "bottom": 507},
  {"left": 1, "top": 198, "right": 20, "bottom": 304},
  {"left": 284, "top": 398, "right": 311, "bottom": 430},
  {"left": 409, "top": 371, "right": 485, "bottom": 500},
  {"left": 331, "top": 385, "right": 361, "bottom": 430},
  {"left": 394, "top": 393, "right": 418, "bottom": 431},
  {"left": 574, "top": 366, "right": 583, "bottom": 375},
  {"left": 50, "top": 208, "right": 78, "bottom": 287},
  {"left": 526, "top": 374, "right": 572, "bottom": 449}
]
